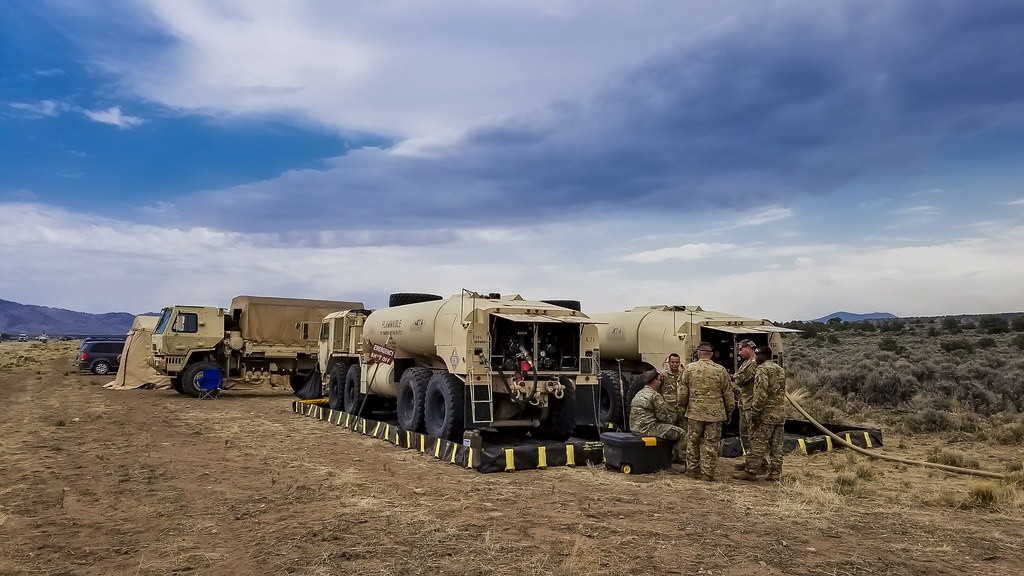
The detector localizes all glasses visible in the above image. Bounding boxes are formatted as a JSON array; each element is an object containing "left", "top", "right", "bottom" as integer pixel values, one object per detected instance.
[{"left": 656, "top": 378, "right": 662, "bottom": 382}]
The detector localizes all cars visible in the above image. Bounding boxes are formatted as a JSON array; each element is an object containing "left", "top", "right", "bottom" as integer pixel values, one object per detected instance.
[
  {"left": 79, "top": 337, "right": 127, "bottom": 350},
  {"left": 78, "top": 341, "right": 125, "bottom": 375}
]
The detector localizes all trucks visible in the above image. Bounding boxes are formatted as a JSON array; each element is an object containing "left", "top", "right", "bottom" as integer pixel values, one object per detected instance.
[{"left": 146, "top": 295, "right": 365, "bottom": 398}]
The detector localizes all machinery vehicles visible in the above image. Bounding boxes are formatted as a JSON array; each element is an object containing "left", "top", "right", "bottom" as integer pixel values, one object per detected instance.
[
  {"left": 316, "top": 288, "right": 601, "bottom": 440},
  {"left": 586, "top": 305, "right": 804, "bottom": 432}
]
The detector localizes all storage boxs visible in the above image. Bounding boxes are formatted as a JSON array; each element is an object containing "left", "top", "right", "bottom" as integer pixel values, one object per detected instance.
[{"left": 599, "top": 431, "right": 673, "bottom": 474}]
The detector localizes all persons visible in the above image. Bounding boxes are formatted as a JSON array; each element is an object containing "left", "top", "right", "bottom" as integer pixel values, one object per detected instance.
[
  {"left": 731, "top": 338, "right": 760, "bottom": 470},
  {"left": 676, "top": 341, "right": 735, "bottom": 480},
  {"left": 629, "top": 369, "right": 687, "bottom": 462},
  {"left": 734, "top": 347, "right": 787, "bottom": 480},
  {"left": 660, "top": 353, "right": 682, "bottom": 466}
]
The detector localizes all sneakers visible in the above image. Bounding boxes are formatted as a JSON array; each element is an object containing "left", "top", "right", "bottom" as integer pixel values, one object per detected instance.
[
  {"left": 764, "top": 473, "right": 777, "bottom": 481},
  {"left": 734, "top": 470, "right": 756, "bottom": 480},
  {"left": 735, "top": 463, "right": 746, "bottom": 470}
]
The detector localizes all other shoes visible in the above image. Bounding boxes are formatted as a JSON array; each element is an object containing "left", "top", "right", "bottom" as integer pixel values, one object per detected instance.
[
  {"left": 672, "top": 455, "right": 685, "bottom": 465},
  {"left": 701, "top": 474, "right": 714, "bottom": 481},
  {"left": 688, "top": 471, "right": 700, "bottom": 479}
]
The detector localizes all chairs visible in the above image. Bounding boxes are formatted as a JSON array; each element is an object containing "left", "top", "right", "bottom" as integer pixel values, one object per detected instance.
[{"left": 195, "top": 369, "right": 223, "bottom": 400}]
